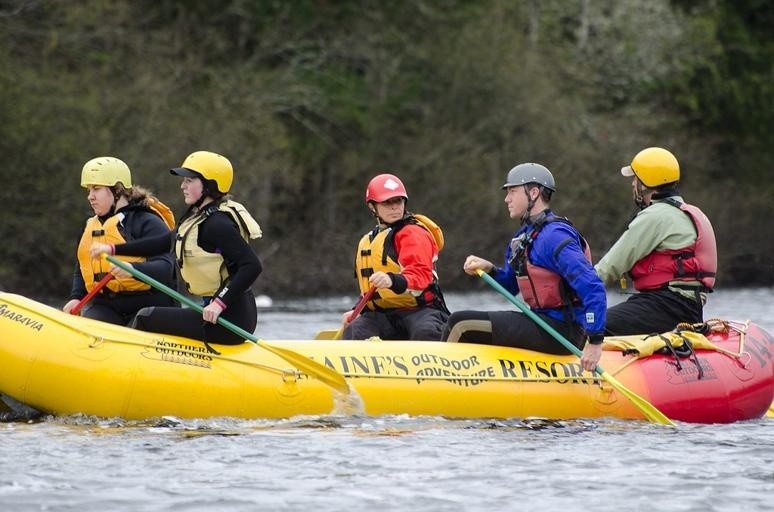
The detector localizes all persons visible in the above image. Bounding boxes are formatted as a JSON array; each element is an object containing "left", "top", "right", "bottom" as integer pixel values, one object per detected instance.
[
  {"left": 342, "top": 173, "right": 451, "bottom": 341},
  {"left": 61, "top": 155, "right": 177, "bottom": 326},
  {"left": 438, "top": 162, "right": 607, "bottom": 375},
  {"left": 88, "top": 151, "right": 264, "bottom": 345},
  {"left": 592, "top": 147, "right": 719, "bottom": 336}
]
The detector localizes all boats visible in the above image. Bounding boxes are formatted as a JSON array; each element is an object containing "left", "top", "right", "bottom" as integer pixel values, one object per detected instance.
[{"left": 0, "top": 291, "right": 774, "bottom": 426}]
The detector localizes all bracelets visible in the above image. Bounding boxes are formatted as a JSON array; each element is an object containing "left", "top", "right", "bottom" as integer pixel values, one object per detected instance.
[
  {"left": 213, "top": 300, "right": 228, "bottom": 313},
  {"left": 108, "top": 244, "right": 115, "bottom": 256}
]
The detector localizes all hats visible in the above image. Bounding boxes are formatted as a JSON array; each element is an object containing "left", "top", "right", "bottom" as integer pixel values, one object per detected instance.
[
  {"left": 169, "top": 168, "right": 200, "bottom": 178},
  {"left": 621, "top": 166, "right": 636, "bottom": 176}
]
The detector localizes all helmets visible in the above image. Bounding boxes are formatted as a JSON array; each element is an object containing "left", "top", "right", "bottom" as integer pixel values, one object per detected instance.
[
  {"left": 502, "top": 162, "right": 555, "bottom": 192},
  {"left": 180, "top": 151, "right": 234, "bottom": 193},
  {"left": 367, "top": 173, "right": 408, "bottom": 201},
  {"left": 632, "top": 147, "right": 681, "bottom": 187},
  {"left": 81, "top": 157, "right": 132, "bottom": 189}
]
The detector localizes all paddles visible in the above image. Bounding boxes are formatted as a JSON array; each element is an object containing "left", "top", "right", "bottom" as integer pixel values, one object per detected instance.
[
  {"left": 101, "top": 253, "right": 351, "bottom": 396},
  {"left": 474, "top": 268, "right": 676, "bottom": 430},
  {"left": 315, "top": 285, "right": 378, "bottom": 340}
]
